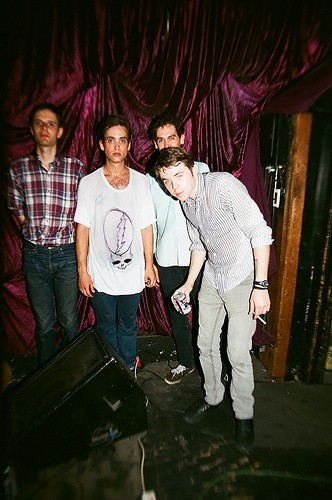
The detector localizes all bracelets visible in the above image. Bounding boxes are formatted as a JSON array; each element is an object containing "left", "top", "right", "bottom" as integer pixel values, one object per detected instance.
[{"left": 253, "top": 286, "right": 268, "bottom": 290}]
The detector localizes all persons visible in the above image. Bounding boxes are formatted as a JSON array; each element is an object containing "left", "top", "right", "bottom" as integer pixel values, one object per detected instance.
[
  {"left": 73, "top": 114, "right": 158, "bottom": 385},
  {"left": 5, "top": 102, "right": 87, "bottom": 368},
  {"left": 148, "top": 147, "right": 274, "bottom": 448},
  {"left": 145, "top": 114, "right": 210, "bottom": 385}
]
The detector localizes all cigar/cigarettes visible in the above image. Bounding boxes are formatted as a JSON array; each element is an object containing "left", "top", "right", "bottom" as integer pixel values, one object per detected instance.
[{"left": 253, "top": 313, "right": 266, "bottom": 324}]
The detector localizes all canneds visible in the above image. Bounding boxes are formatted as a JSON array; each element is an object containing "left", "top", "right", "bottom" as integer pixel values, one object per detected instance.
[{"left": 173, "top": 292, "right": 191, "bottom": 315}]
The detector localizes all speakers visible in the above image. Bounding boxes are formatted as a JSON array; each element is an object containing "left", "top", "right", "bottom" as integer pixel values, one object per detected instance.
[
  {"left": 1, "top": 325, "right": 148, "bottom": 471},
  {"left": 135, "top": 335, "right": 178, "bottom": 364}
]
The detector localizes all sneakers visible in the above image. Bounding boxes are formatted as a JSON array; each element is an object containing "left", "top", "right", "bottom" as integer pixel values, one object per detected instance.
[{"left": 165, "top": 364, "right": 195, "bottom": 385}]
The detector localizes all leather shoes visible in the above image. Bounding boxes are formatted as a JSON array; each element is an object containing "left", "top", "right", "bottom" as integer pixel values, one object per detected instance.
[
  {"left": 183, "top": 398, "right": 223, "bottom": 422},
  {"left": 235, "top": 419, "right": 254, "bottom": 452}
]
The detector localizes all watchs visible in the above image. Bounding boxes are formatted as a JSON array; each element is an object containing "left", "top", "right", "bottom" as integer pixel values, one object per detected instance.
[{"left": 254, "top": 279, "right": 269, "bottom": 287}]
